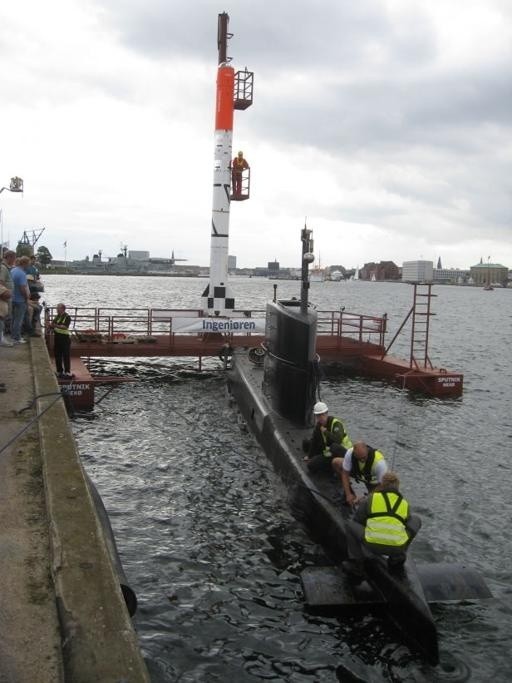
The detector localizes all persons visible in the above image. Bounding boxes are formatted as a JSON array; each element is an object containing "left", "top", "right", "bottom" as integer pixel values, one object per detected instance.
[
  {"left": 346, "top": 473, "right": 422, "bottom": 569},
  {"left": 231, "top": 151, "right": 250, "bottom": 200},
  {"left": 0, "top": 249, "right": 44, "bottom": 345},
  {"left": 330, "top": 439, "right": 389, "bottom": 504},
  {"left": 48, "top": 302, "right": 71, "bottom": 380},
  {"left": 302, "top": 402, "right": 353, "bottom": 479}
]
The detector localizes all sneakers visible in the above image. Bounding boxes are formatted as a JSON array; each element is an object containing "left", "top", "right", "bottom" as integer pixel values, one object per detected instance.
[
  {"left": 0, "top": 332, "right": 40, "bottom": 348},
  {"left": 388, "top": 553, "right": 407, "bottom": 567},
  {"left": 55, "top": 371, "right": 73, "bottom": 378},
  {"left": 341, "top": 560, "right": 363, "bottom": 577}
]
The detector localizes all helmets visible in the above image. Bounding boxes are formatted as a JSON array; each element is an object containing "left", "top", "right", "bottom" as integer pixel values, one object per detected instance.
[{"left": 313, "top": 402, "right": 329, "bottom": 415}]
